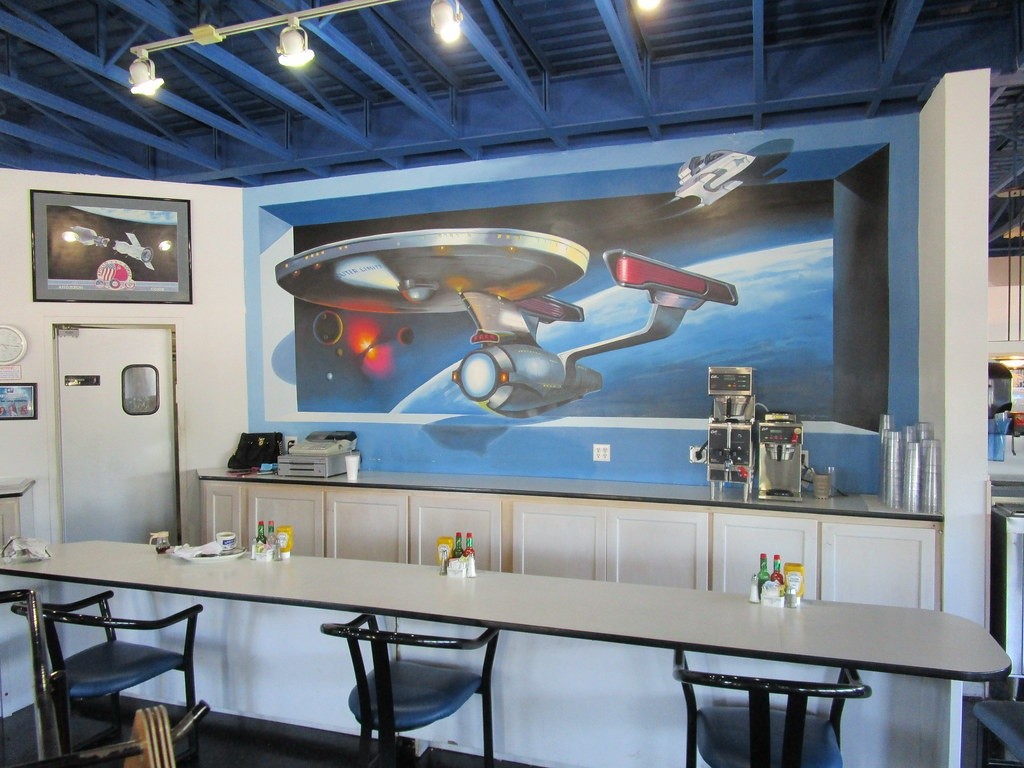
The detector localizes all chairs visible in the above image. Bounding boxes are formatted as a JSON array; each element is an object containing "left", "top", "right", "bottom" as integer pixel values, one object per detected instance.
[
  {"left": 321, "top": 613, "right": 499, "bottom": 768},
  {"left": 673, "top": 649, "right": 873, "bottom": 768},
  {"left": 972, "top": 700, "right": 1024, "bottom": 768},
  {"left": 0, "top": 588, "right": 210, "bottom": 768}
]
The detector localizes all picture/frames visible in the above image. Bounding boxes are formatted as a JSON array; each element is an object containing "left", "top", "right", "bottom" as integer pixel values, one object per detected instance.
[
  {"left": 30, "top": 189, "right": 194, "bottom": 305},
  {"left": 0, "top": 383, "right": 35, "bottom": 420}
]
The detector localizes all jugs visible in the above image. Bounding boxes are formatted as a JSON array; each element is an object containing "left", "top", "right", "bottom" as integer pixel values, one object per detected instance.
[
  {"left": 988, "top": 418, "right": 1012, "bottom": 461},
  {"left": 149, "top": 530, "right": 170, "bottom": 554}
]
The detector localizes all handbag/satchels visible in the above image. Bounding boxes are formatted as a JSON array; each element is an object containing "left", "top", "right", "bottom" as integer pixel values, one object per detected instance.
[{"left": 228, "top": 432, "right": 283, "bottom": 469}]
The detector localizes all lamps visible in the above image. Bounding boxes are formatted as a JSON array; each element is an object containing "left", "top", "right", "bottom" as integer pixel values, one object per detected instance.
[
  {"left": 130, "top": 49, "right": 165, "bottom": 96},
  {"left": 432, "top": 1, "right": 464, "bottom": 44},
  {"left": 276, "top": 17, "right": 315, "bottom": 66}
]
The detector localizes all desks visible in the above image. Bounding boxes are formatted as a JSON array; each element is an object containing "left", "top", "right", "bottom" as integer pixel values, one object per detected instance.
[{"left": 1, "top": 537, "right": 1013, "bottom": 768}]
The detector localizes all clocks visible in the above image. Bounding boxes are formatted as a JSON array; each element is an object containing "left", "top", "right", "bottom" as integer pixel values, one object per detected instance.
[{"left": 0, "top": 325, "right": 28, "bottom": 365}]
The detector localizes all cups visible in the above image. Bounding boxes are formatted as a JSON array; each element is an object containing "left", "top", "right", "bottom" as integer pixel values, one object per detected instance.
[
  {"left": 345, "top": 455, "right": 360, "bottom": 480},
  {"left": 878, "top": 413, "right": 941, "bottom": 515},
  {"left": 824, "top": 466, "right": 838, "bottom": 496},
  {"left": 813, "top": 473, "right": 831, "bottom": 499},
  {"left": 216, "top": 532, "right": 238, "bottom": 550}
]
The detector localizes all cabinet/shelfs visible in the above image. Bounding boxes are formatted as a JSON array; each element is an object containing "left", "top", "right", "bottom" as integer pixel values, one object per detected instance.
[
  {"left": 0, "top": 477, "right": 36, "bottom": 546},
  {"left": 193, "top": 483, "right": 938, "bottom": 613}
]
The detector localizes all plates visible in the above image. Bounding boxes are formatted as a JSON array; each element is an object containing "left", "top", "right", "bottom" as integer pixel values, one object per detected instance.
[{"left": 165, "top": 545, "right": 247, "bottom": 563}]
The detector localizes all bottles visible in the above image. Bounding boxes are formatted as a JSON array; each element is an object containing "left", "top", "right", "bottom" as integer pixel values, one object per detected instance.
[
  {"left": 256, "top": 521, "right": 267, "bottom": 544},
  {"left": 770, "top": 555, "right": 783, "bottom": 585},
  {"left": 784, "top": 563, "right": 805, "bottom": 606},
  {"left": 439, "top": 550, "right": 449, "bottom": 575},
  {"left": 786, "top": 587, "right": 797, "bottom": 608},
  {"left": 436, "top": 536, "right": 454, "bottom": 565},
  {"left": 464, "top": 533, "right": 475, "bottom": 559},
  {"left": 251, "top": 537, "right": 256, "bottom": 559},
  {"left": 267, "top": 520, "right": 276, "bottom": 544},
  {"left": 452, "top": 531, "right": 463, "bottom": 558},
  {"left": 749, "top": 574, "right": 760, "bottom": 603},
  {"left": 274, "top": 538, "right": 281, "bottom": 561},
  {"left": 467, "top": 554, "right": 476, "bottom": 578},
  {"left": 277, "top": 526, "right": 293, "bottom": 558},
  {"left": 757, "top": 554, "right": 770, "bottom": 600}
]
